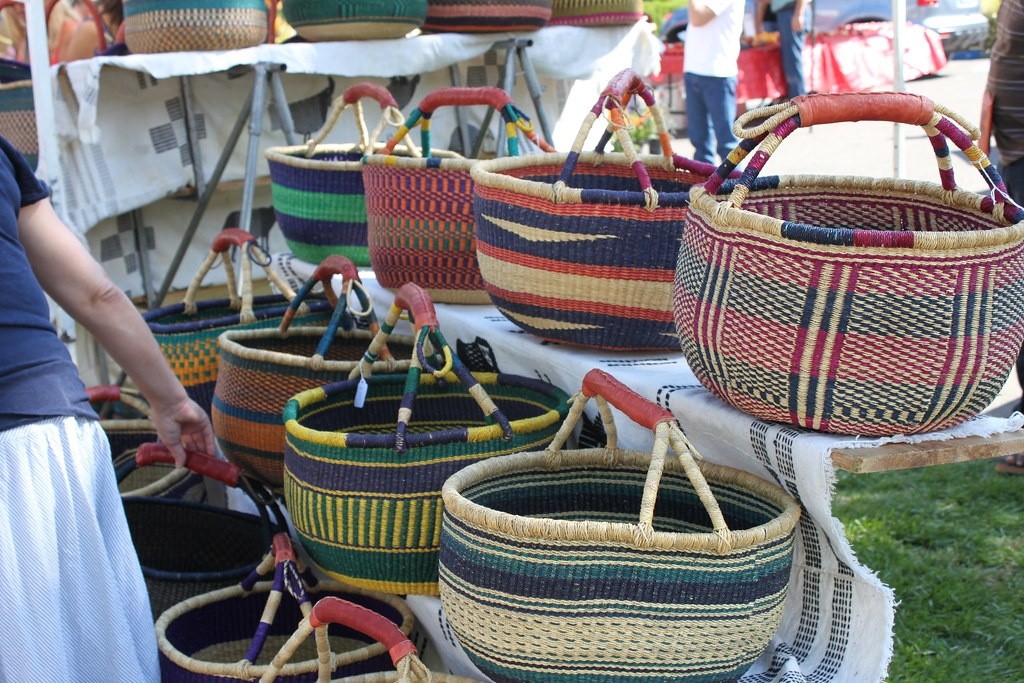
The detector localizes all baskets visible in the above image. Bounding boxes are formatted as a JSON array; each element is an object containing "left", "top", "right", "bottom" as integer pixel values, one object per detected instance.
[{"left": 0, "top": 0, "right": 1024, "bottom": 683}]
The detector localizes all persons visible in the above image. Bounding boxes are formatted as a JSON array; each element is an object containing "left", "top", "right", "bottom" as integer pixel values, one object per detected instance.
[
  {"left": 684, "top": 0, "right": 746, "bottom": 167},
  {"left": 0, "top": 136, "right": 216, "bottom": 682},
  {"left": 980, "top": 1, "right": 1023, "bottom": 472},
  {"left": 755, "top": 0, "right": 811, "bottom": 99}
]
